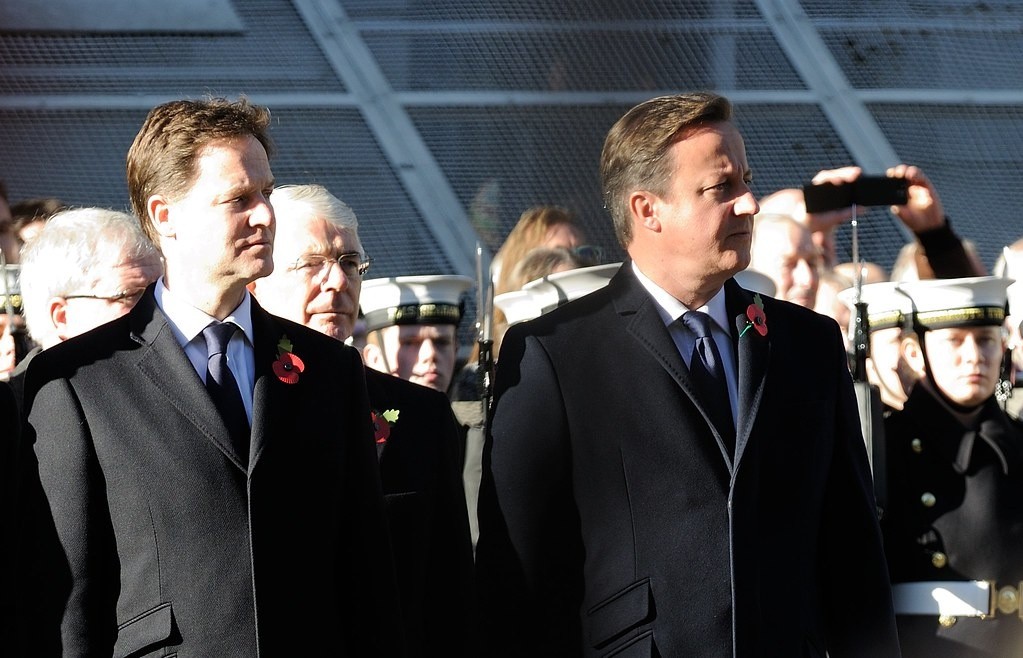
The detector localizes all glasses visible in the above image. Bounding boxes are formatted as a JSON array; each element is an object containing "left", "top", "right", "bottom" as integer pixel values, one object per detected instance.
[
  {"left": 61, "top": 292, "right": 142, "bottom": 308},
  {"left": 269, "top": 253, "right": 369, "bottom": 278}
]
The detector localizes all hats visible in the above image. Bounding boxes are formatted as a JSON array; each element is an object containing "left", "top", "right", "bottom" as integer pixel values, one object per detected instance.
[
  {"left": 521, "top": 259, "right": 623, "bottom": 314},
  {"left": 492, "top": 291, "right": 527, "bottom": 327},
  {"left": 836, "top": 282, "right": 903, "bottom": 334},
  {"left": 358, "top": 274, "right": 476, "bottom": 333},
  {"left": 0, "top": 265, "right": 22, "bottom": 314},
  {"left": 895, "top": 276, "right": 1017, "bottom": 332}
]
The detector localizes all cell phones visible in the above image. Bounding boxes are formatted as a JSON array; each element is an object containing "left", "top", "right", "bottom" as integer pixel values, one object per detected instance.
[{"left": 802, "top": 174, "right": 908, "bottom": 214}]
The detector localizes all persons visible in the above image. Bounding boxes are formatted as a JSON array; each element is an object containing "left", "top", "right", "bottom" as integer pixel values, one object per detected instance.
[
  {"left": 743, "top": 160, "right": 1022, "bottom": 658},
  {"left": 0, "top": 183, "right": 165, "bottom": 386},
  {"left": 472, "top": 85, "right": 902, "bottom": 657},
  {"left": 0, "top": 97, "right": 411, "bottom": 658},
  {"left": 254, "top": 180, "right": 623, "bottom": 657}
]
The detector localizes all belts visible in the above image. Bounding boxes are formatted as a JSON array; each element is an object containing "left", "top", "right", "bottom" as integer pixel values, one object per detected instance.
[{"left": 889, "top": 577, "right": 1023, "bottom": 621}]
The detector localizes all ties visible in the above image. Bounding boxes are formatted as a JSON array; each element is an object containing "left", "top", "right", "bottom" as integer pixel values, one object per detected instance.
[
  {"left": 678, "top": 310, "right": 737, "bottom": 465},
  {"left": 200, "top": 324, "right": 251, "bottom": 466}
]
